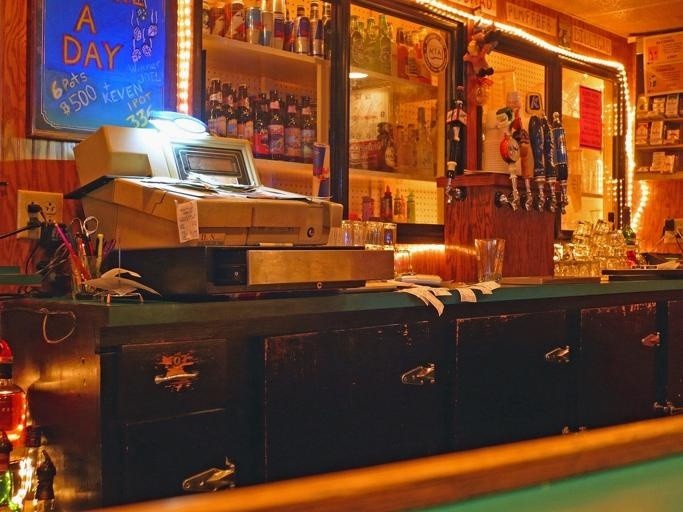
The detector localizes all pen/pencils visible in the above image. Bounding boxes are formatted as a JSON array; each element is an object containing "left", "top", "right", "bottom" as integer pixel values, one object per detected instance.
[{"left": 54, "top": 222, "right": 117, "bottom": 283}]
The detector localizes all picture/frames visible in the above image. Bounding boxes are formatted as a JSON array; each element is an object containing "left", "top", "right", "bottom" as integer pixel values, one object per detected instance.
[{"left": 26, "top": 1, "right": 177, "bottom": 141}]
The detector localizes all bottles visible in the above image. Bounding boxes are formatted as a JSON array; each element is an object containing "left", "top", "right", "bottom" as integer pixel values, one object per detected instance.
[
  {"left": 212, "top": 2, "right": 226, "bottom": 37},
  {"left": 364, "top": 17, "right": 379, "bottom": 72},
  {"left": 226, "top": 0, "right": 246, "bottom": 42},
  {"left": 308, "top": 1, "right": 324, "bottom": 60},
  {"left": 406, "top": 187, "right": 416, "bottom": 223},
  {"left": 350, "top": 16, "right": 366, "bottom": 68},
  {"left": 245, "top": 0, "right": 261, "bottom": 44},
  {"left": 12, "top": 424, "right": 50, "bottom": 508},
  {"left": 376, "top": 15, "right": 392, "bottom": 76},
  {"left": 294, "top": 5, "right": 311, "bottom": 56},
  {"left": 368, "top": 122, "right": 396, "bottom": 171},
  {"left": 284, "top": 95, "right": 302, "bottom": 162},
  {"left": 202, "top": 1, "right": 211, "bottom": 34},
  {"left": 300, "top": 95, "right": 316, "bottom": 162},
  {"left": 401, "top": 195, "right": 407, "bottom": 223},
  {"left": 260, "top": 1, "right": 273, "bottom": 46},
  {"left": 446, "top": 86, "right": 465, "bottom": 180},
  {"left": 236, "top": 84, "right": 253, "bottom": 139},
  {"left": 428, "top": 106, "right": 437, "bottom": 176},
  {"left": 396, "top": 24, "right": 433, "bottom": 83},
  {"left": 358, "top": 21, "right": 365, "bottom": 35},
  {"left": 0, "top": 441, "right": 16, "bottom": 512},
  {"left": 268, "top": 89, "right": 284, "bottom": 159},
  {"left": 321, "top": 0, "right": 332, "bottom": 60},
  {"left": 622, "top": 206, "right": 637, "bottom": 265},
  {"left": 254, "top": 93, "right": 270, "bottom": 153},
  {"left": 607, "top": 212, "right": 617, "bottom": 240},
  {"left": 407, "top": 123, "right": 418, "bottom": 168},
  {"left": 395, "top": 125, "right": 416, "bottom": 169},
  {"left": 0, "top": 339, "right": 26, "bottom": 448},
  {"left": 394, "top": 188, "right": 402, "bottom": 223},
  {"left": 283, "top": 0, "right": 294, "bottom": 52},
  {"left": 386, "top": 22, "right": 393, "bottom": 41},
  {"left": 412, "top": 107, "right": 434, "bottom": 179},
  {"left": 380, "top": 184, "right": 393, "bottom": 222},
  {"left": 273, "top": 1, "right": 284, "bottom": 50},
  {"left": 222, "top": 82, "right": 237, "bottom": 138},
  {"left": 207, "top": 77, "right": 226, "bottom": 136}
]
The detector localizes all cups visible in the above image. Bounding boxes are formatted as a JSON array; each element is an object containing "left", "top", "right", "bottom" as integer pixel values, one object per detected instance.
[
  {"left": 475, "top": 238, "right": 505, "bottom": 284},
  {"left": 383, "top": 223, "right": 397, "bottom": 250},
  {"left": 363, "top": 221, "right": 384, "bottom": 250},
  {"left": 312, "top": 141, "right": 326, "bottom": 178},
  {"left": 341, "top": 220, "right": 363, "bottom": 246},
  {"left": 70, "top": 254, "right": 102, "bottom": 302},
  {"left": 554, "top": 219, "right": 628, "bottom": 280}
]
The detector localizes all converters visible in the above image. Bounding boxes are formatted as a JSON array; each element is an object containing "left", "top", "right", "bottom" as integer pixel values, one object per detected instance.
[
  {"left": 41, "top": 222, "right": 66, "bottom": 249},
  {"left": 663, "top": 218, "right": 674, "bottom": 231}
]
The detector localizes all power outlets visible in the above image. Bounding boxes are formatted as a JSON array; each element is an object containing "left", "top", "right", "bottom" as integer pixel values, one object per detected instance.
[{"left": 17, "top": 189, "right": 64, "bottom": 240}]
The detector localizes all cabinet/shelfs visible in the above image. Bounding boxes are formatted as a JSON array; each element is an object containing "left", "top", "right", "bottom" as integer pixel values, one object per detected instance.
[
  {"left": 0, "top": 268, "right": 683, "bottom": 506},
  {"left": 190, "top": 1, "right": 624, "bottom": 243},
  {"left": 629, "top": 33, "right": 682, "bottom": 179}
]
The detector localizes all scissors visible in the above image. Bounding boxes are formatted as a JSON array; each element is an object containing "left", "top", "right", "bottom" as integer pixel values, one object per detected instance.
[{"left": 70, "top": 216, "right": 98, "bottom": 239}]
